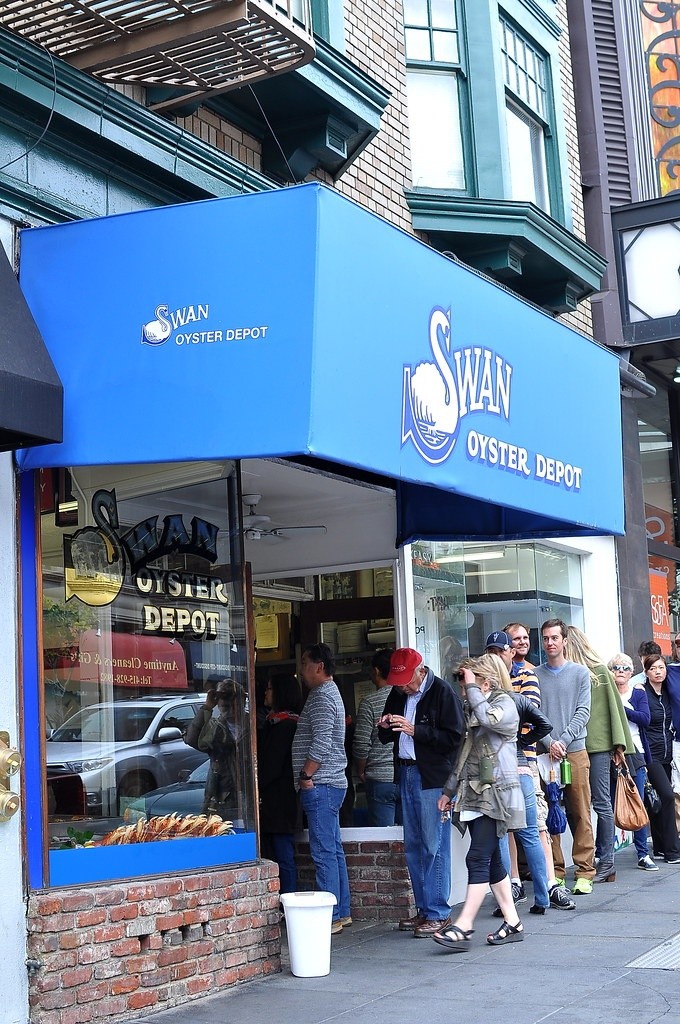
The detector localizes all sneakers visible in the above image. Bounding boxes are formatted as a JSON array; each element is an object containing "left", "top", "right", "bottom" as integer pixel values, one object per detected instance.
[
  {"left": 497, "top": 881, "right": 527, "bottom": 912},
  {"left": 555, "top": 877, "right": 566, "bottom": 891},
  {"left": 548, "top": 884, "right": 577, "bottom": 909},
  {"left": 637, "top": 854, "right": 659, "bottom": 871},
  {"left": 572, "top": 878, "right": 593, "bottom": 894}
]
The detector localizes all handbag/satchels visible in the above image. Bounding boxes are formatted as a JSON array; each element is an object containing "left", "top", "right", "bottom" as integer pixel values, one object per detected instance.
[
  {"left": 643, "top": 779, "right": 663, "bottom": 814},
  {"left": 614, "top": 749, "right": 650, "bottom": 831}
]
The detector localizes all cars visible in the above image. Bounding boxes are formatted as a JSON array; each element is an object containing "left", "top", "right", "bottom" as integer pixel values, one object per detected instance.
[{"left": 122, "top": 758, "right": 211, "bottom": 825}]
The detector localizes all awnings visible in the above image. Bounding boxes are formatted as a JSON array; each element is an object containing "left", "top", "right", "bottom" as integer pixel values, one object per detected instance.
[{"left": 43, "top": 627, "right": 247, "bottom": 687}]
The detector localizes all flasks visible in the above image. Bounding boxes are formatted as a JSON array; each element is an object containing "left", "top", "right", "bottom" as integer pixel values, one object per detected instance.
[{"left": 560, "top": 757, "right": 572, "bottom": 784}]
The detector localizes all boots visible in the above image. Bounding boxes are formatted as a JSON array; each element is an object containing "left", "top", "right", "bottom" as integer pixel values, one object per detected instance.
[{"left": 593, "top": 854, "right": 616, "bottom": 882}]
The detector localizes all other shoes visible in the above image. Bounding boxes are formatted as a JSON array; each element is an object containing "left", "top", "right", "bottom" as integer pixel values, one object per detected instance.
[
  {"left": 331, "top": 921, "right": 343, "bottom": 935},
  {"left": 530, "top": 903, "right": 545, "bottom": 915},
  {"left": 340, "top": 916, "right": 352, "bottom": 927},
  {"left": 492, "top": 908, "right": 503, "bottom": 917},
  {"left": 664, "top": 856, "right": 680, "bottom": 864},
  {"left": 653, "top": 850, "right": 666, "bottom": 859}
]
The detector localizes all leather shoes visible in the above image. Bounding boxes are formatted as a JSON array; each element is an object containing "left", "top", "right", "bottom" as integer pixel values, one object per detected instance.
[
  {"left": 415, "top": 917, "right": 452, "bottom": 938},
  {"left": 399, "top": 913, "right": 427, "bottom": 930}
]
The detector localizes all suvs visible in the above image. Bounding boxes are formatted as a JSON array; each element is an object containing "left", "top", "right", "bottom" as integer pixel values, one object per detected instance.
[{"left": 46, "top": 693, "right": 249, "bottom": 818}]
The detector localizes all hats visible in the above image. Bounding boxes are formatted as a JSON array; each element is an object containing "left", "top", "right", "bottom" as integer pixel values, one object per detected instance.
[
  {"left": 386, "top": 648, "right": 423, "bottom": 686},
  {"left": 484, "top": 630, "right": 512, "bottom": 651}
]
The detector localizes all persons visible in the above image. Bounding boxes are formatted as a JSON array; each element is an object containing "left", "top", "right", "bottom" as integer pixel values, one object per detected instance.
[
  {"left": 185, "top": 679, "right": 253, "bottom": 827},
  {"left": 439, "top": 637, "right": 465, "bottom": 694},
  {"left": 257, "top": 619, "right": 680, "bottom": 951}
]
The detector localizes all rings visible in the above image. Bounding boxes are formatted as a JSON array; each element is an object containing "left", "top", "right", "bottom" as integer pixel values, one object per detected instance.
[{"left": 399, "top": 724, "right": 403, "bottom": 728}]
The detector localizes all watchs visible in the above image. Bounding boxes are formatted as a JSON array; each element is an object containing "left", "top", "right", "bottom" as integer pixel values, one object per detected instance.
[{"left": 299, "top": 770, "right": 312, "bottom": 780}]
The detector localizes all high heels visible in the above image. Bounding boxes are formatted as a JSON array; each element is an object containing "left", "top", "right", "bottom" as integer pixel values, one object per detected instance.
[
  {"left": 433, "top": 924, "right": 474, "bottom": 950},
  {"left": 487, "top": 921, "right": 525, "bottom": 945}
]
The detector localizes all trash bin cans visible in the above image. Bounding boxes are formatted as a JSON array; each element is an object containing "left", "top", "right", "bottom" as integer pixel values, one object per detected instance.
[{"left": 280, "top": 891, "right": 337, "bottom": 979}]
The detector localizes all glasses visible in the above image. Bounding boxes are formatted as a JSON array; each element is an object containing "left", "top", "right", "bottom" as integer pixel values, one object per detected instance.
[
  {"left": 221, "top": 691, "right": 245, "bottom": 700},
  {"left": 613, "top": 665, "right": 630, "bottom": 671},
  {"left": 313, "top": 642, "right": 322, "bottom": 658}
]
